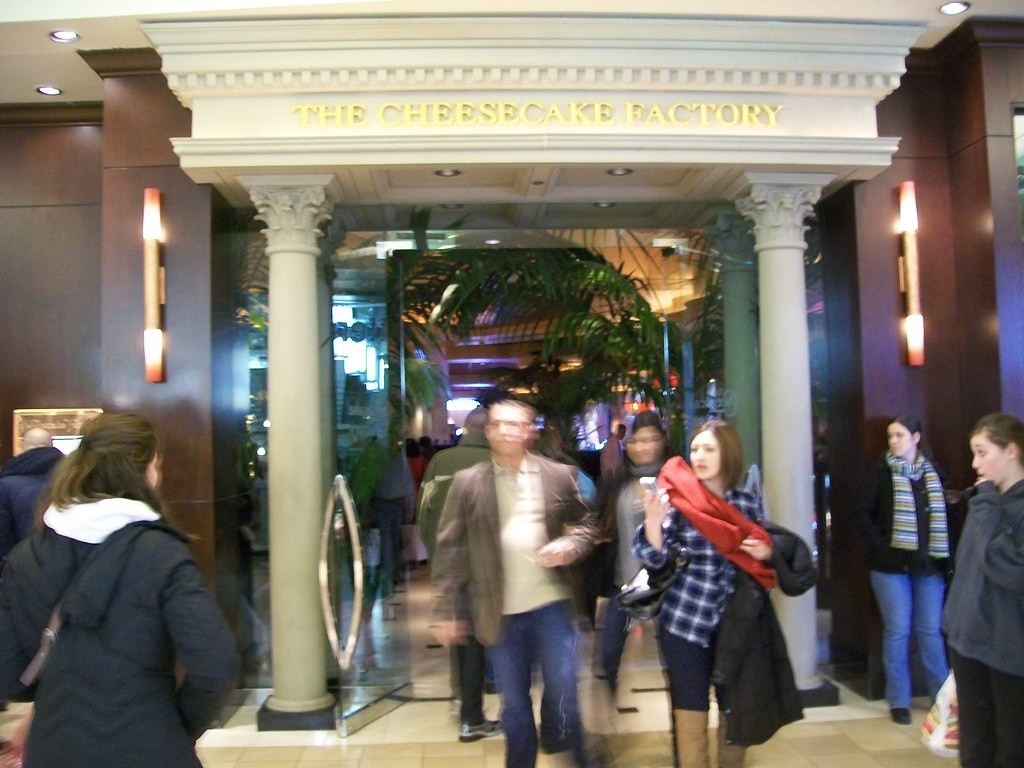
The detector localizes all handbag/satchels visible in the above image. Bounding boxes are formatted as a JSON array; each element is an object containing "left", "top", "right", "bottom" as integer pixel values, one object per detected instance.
[
  {"left": 919, "top": 671, "right": 961, "bottom": 758},
  {"left": 615, "top": 533, "right": 694, "bottom": 622}
]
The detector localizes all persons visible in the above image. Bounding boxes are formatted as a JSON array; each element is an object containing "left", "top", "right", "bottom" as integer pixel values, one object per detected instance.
[
  {"left": 590, "top": 411, "right": 681, "bottom": 768},
  {"left": 848, "top": 414, "right": 953, "bottom": 725},
  {"left": 0, "top": 411, "right": 236, "bottom": 768},
  {"left": 614, "top": 421, "right": 776, "bottom": 768},
  {"left": 939, "top": 414, "right": 1024, "bottom": 768},
  {"left": 429, "top": 396, "right": 602, "bottom": 768},
  {"left": 367, "top": 409, "right": 627, "bottom": 741},
  {"left": 0, "top": 428, "right": 68, "bottom": 556}
]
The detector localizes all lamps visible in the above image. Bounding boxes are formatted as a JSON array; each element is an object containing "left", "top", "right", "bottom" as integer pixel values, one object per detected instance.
[
  {"left": 139, "top": 187, "right": 167, "bottom": 384},
  {"left": 898, "top": 181, "right": 926, "bottom": 368}
]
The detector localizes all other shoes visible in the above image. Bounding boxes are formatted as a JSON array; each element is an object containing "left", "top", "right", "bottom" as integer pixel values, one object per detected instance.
[{"left": 890, "top": 707, "right": 911, "bottom": 725}]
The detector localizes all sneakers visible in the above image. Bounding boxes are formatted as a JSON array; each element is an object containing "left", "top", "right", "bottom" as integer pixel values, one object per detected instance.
[{"left": 459, "top": 719, "right": 503, "bottom": 743}]
